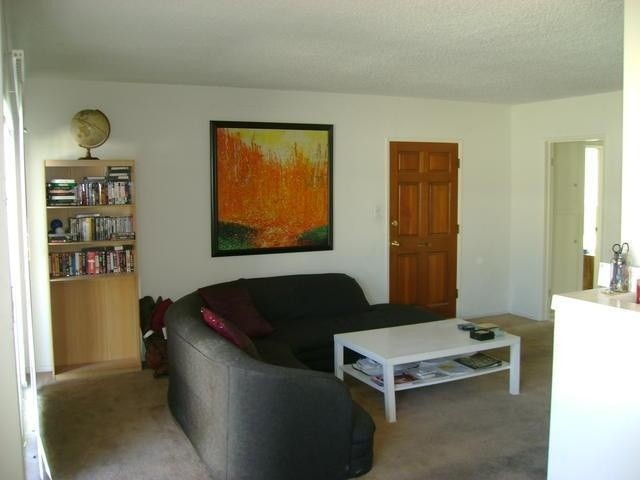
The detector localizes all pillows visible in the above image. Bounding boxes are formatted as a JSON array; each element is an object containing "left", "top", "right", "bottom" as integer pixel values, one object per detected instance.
[{"left": 198, "top": 281, "right": 274, "bottom": 363}]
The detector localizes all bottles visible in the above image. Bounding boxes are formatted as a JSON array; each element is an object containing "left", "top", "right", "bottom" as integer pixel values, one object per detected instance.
[{"left": 610, "top": 264, "right": 629, "bottom": 293}]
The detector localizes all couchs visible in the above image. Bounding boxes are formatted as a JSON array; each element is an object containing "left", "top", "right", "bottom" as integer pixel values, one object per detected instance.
[{"left": 164, "top": 273, "right": 446, "bottom": 480}]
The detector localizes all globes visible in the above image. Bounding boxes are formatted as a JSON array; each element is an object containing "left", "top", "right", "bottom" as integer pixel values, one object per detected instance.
[{"left": 70, "top": 109, "right": 111, "bottom": 160}]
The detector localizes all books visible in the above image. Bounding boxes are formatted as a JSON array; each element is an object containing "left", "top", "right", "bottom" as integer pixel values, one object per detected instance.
[
  {"left": 352, "top": 354, "right": 504, "bottom": 389},
  {"left": 46, "top": 165, "right": 135, "bottom": 278}
]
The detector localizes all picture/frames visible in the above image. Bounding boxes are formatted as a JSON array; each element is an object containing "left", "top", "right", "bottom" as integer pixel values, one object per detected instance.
[{"left": 208, "top": 118, "right": 333, "bottom": 258}]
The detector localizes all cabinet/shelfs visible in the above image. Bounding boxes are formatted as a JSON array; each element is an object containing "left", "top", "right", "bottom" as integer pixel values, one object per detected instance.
[{"left": 44, "top": 159, "right": 142, "bottom": 382}]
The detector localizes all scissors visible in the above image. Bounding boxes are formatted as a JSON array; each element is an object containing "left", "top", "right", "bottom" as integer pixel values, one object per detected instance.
[{"left": 612, "top": 242, "right": 629, "bottom": 254}]
